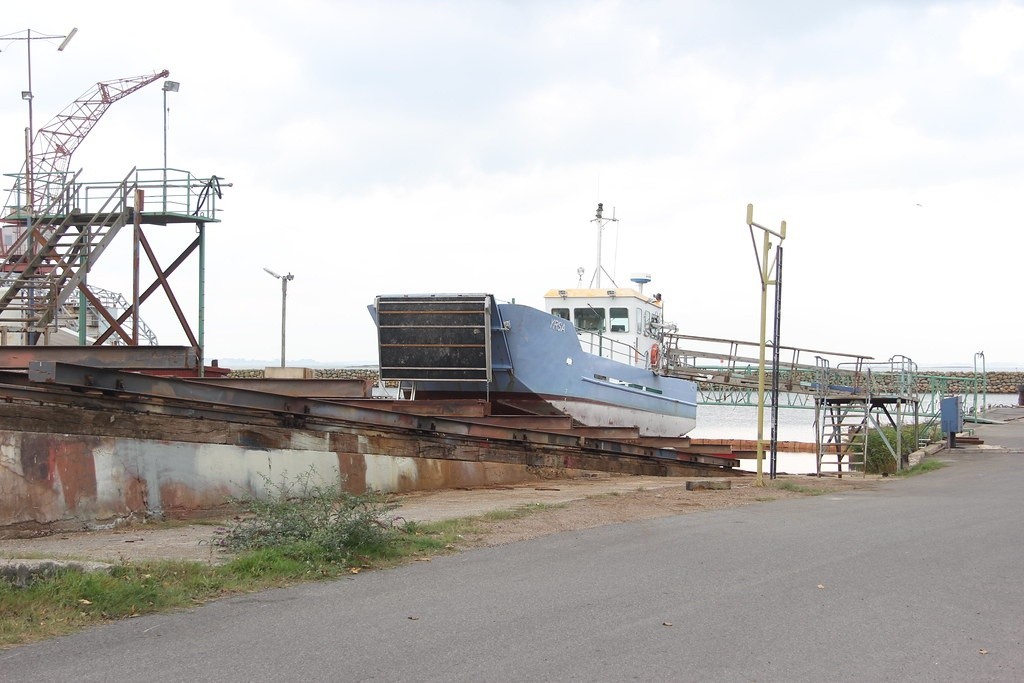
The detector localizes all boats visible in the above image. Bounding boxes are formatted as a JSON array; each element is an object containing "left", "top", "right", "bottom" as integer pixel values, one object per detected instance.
[{"left": 366, "top": 202, "right": 698, "bottom": 436}]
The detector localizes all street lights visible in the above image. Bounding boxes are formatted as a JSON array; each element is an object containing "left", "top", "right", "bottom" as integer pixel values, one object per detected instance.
[{"left": 162, "top": 80, "right": 180, "bottom": 213}]
